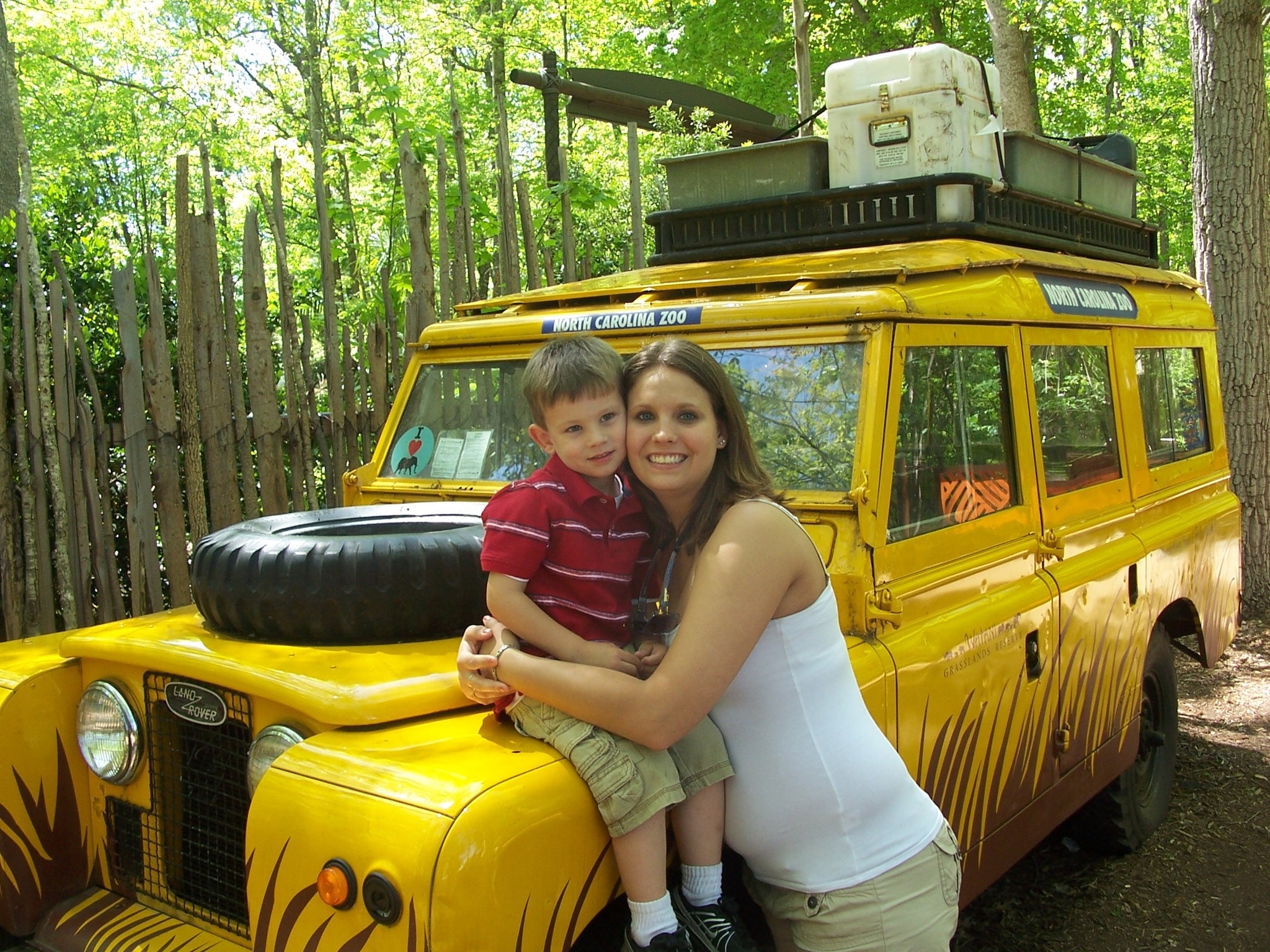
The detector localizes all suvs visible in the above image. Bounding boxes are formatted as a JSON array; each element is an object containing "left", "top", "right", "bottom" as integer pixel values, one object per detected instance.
[{"left": 1, "top": 173, "right": 1245, "bottom": 952}]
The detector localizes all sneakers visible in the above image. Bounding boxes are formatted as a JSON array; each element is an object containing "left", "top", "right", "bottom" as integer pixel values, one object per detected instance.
[
  {"left": 670, "top": 884, "right": 758, "bottom": 952},
  {"left": 621, "top": 910, "right": 694, "bottom": 952}
]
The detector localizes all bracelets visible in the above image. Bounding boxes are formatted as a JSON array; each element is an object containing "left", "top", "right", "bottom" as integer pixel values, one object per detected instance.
[{"left": 491, "top": 644, "right": 518, "bottom": 682}]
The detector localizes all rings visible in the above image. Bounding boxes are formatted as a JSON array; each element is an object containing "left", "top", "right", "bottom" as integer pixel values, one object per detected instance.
[
  {"left": 468, "top": 674, "right": 479, "bottom": 688},
  {"left": 471, "top": 689, "right": 476, "bottom": 698}
]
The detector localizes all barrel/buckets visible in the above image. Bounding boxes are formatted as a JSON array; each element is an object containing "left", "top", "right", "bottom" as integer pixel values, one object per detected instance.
[{"left": 824, "top": 42, "right": 1005, "bottom": 223}]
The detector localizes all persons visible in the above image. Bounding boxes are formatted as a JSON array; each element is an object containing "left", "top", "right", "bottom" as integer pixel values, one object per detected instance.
[
  {"left": 480, "top": 332, "right": 774, "bottom": 952},
  {"left": 455, "top": 340, "right": 963, "bottom": 952}
]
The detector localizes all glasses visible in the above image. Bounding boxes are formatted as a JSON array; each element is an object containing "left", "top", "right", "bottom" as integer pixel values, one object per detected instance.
[{"left": 624, "top": 526, "right": 691, "bottom": 637}]
